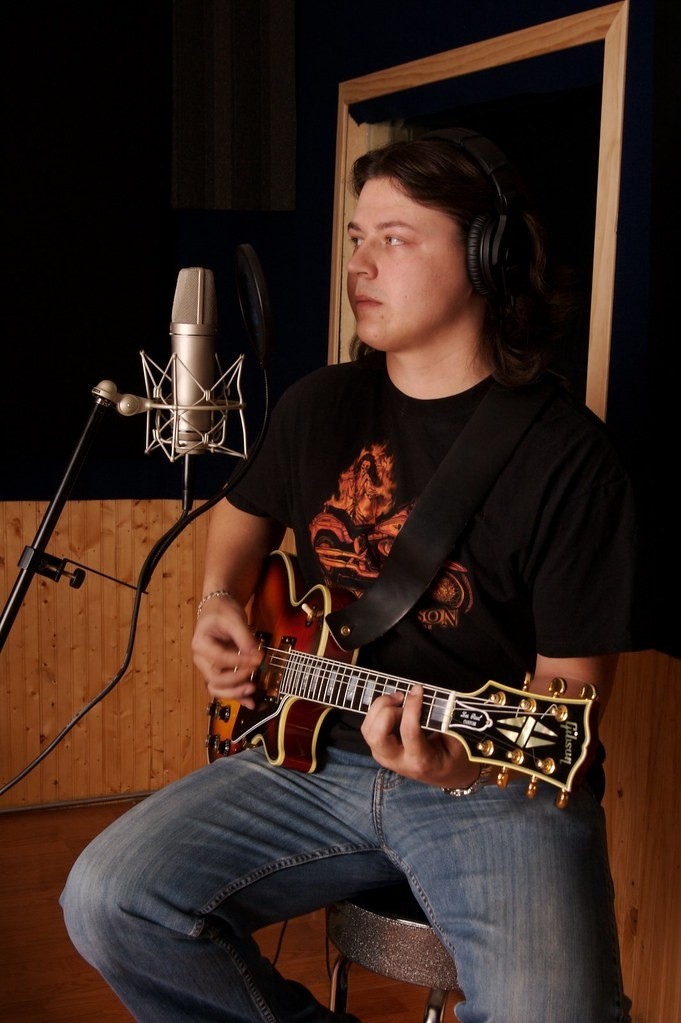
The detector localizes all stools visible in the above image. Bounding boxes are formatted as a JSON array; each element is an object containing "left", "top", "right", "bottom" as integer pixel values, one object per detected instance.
[{"left": 324, "top": 880, "right": 462, "bottom": 1023}]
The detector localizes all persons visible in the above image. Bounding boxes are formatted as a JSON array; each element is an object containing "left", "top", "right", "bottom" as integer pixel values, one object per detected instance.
[{"left": 59, "top": 133, "right": 625, "bottom": 1023}]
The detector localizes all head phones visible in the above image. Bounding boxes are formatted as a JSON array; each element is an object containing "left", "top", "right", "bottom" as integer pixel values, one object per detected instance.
[{"left": 420, "top": 130, "right": 534, "bottom": 302}]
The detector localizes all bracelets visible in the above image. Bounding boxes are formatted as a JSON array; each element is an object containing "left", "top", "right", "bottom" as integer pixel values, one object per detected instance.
[
  {"left": 196, "top": 590, "right": 236, "bottom": 620},
  {"left": 439, "top": 764, "right": 492, "bottom": 798}
]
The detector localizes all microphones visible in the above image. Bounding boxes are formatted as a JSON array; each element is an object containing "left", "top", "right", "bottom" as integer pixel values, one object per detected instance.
[{"left": 173, "top": 266, "right": 217, "bottom": 517}]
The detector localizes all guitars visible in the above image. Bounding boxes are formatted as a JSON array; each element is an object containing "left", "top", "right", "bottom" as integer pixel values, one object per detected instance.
[{"left": 204, "top": 548, "right": 600, "bottom": 808}]
